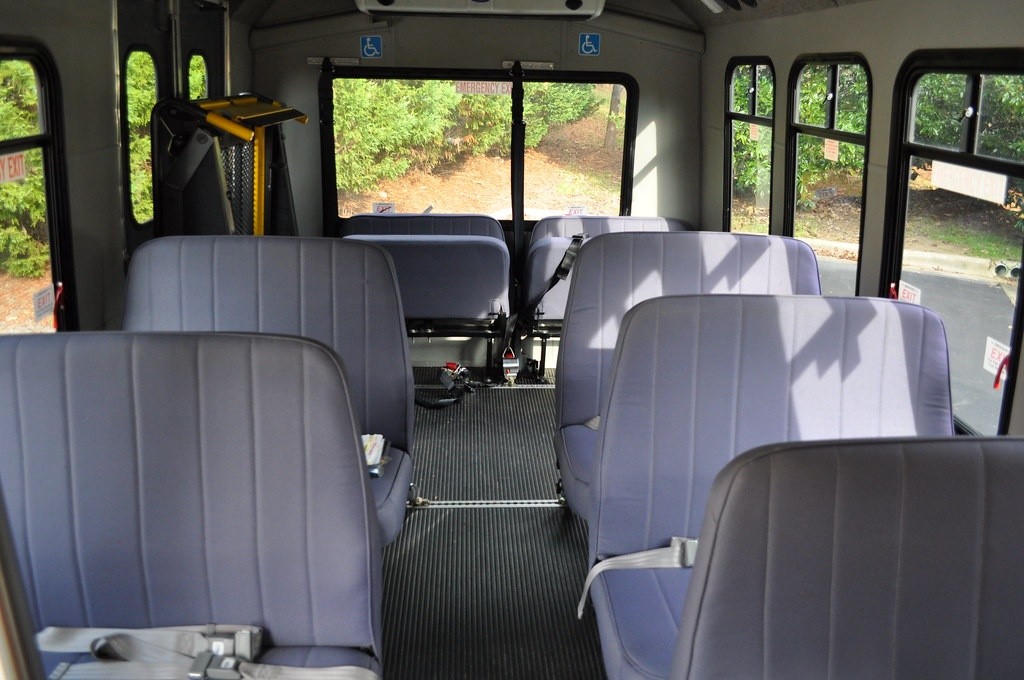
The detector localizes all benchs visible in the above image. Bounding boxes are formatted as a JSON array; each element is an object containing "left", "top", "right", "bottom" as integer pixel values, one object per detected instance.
[
  {"left": 1, "top": 215, "right": 510, "bottom": 680},
  {"left": 523, "top": 216, "right": 1024, "bottom": 680}
]
